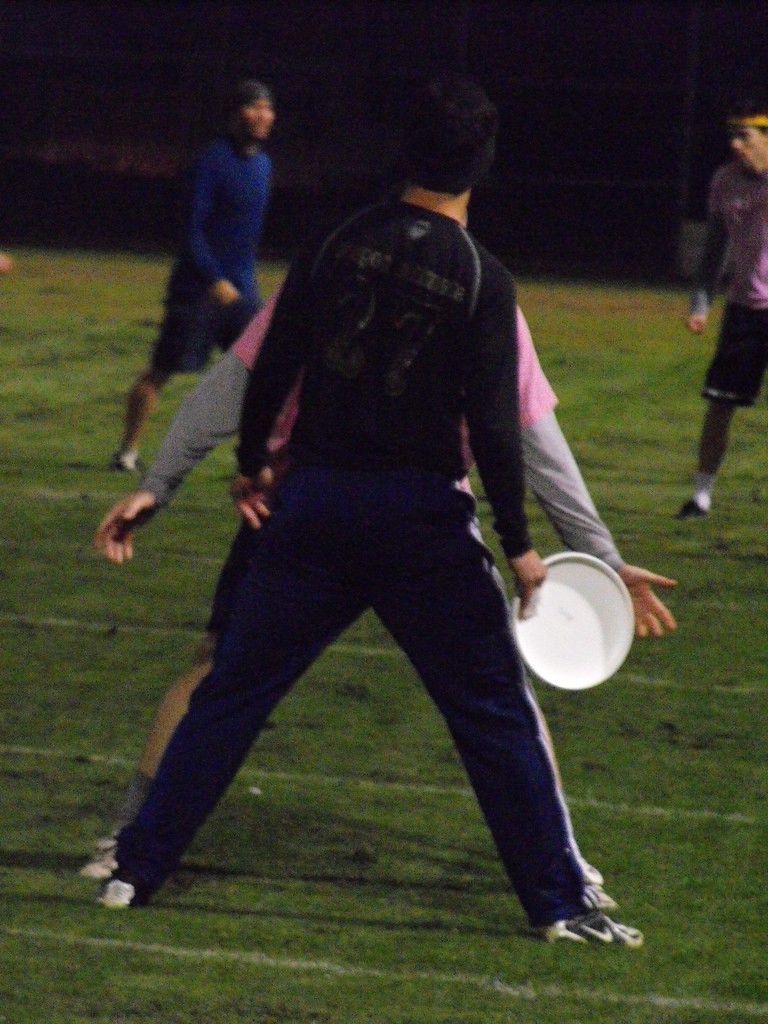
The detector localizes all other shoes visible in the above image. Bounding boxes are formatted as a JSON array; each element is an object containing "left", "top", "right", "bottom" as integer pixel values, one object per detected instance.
[
  {"left": 581, "top": 881, "right": 620, "bottom": 911},
  {"left": 78, "top": 837, "right": 123, "bottom": 879}
]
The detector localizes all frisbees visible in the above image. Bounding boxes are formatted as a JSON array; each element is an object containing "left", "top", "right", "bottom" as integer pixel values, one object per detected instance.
[{"left": 510, "top": 550, "right": 638, "bottom": 693}]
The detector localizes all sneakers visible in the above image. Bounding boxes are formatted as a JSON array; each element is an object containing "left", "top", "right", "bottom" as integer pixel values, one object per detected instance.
[
  {"left": 111, "top": 451, "right": 139, "bottom": 474},
  {"left": 535, "top": 911, "right": 649, "bottom": 950},
  {"left": 682, "top": 493, "right": 712, "bottom": 516},
  {"left": 95, "top": 875, "right": 138, "bottom": 913}
]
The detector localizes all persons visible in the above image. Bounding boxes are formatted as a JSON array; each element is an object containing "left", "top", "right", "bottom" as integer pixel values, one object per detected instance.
[
  {"left": 82, "top": 66, "right": 682, "bottom": 950},
  {"left": 672, "top": 96, "right": 768, "bottom": 514},
  {"left": 109, "top": 78, "right": 279, "bottom": 472}
]
